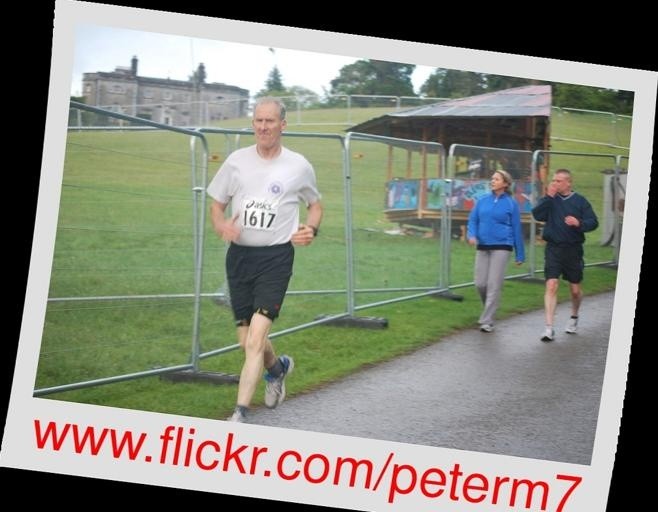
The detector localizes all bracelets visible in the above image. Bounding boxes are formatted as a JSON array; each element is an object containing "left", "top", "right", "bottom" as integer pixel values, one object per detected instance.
[{"left": 309, "top": 224, "right": 318, "bottom": 237}]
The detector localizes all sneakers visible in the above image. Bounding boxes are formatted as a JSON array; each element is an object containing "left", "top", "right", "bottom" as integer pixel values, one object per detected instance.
[
  {"left": 480, "top": 323, "right": 495, "bottom": 332},
  {"left": 262, "top": 354, "right": 295, "bottom": 410},
  {"left": 540, "top": 327, "right": 554, "bottom": 341},
  {"left": 228, "top": 411, "right": 250, "bottom": 423},
  {"left": 565, "top": 318, "right": 578, "bottom": 334}
]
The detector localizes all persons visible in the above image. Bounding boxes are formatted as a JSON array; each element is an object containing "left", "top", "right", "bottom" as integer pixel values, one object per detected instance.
[
  {"left": 466, "top": 170, "right": 525, "bottom": 333},
  {"left": 531, "top": 169, "right": 599, "bottom": 342},
  {"left": 205, "top": 95, "right": 322, "bottom": 423}
]
